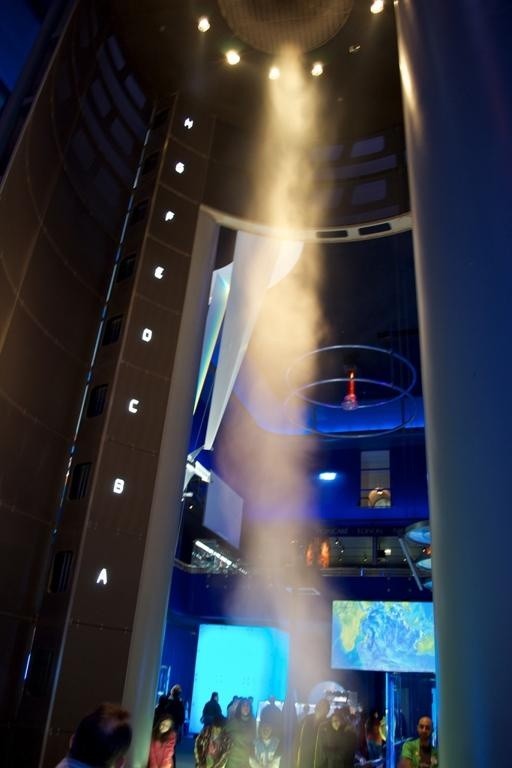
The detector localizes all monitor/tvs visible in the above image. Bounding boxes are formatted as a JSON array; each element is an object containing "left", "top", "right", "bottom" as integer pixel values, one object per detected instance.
[{"left": 202, "top": 467, "right": 246, "bottom": 551}]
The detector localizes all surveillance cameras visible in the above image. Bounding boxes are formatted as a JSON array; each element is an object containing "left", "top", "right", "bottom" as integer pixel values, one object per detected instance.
[{"left": 194, "top": 461, "right": 212, "bottom": 485}]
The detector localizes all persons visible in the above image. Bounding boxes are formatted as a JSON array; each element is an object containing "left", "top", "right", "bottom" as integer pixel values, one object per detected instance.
[
  {"left": 147, "top": 681, "right": 407, "bottom": 767},
  {"left": 53, "top": 701, "right": 134, "bottom": 766},
  {"left": 396, "top": 714, "right": 439, "bottom": 767}
]
